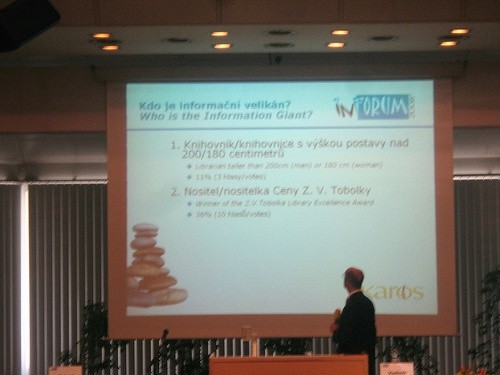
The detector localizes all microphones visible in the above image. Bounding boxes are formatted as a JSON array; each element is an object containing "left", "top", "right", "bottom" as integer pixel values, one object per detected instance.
[{"left": 333, "top": 308, "right": 341, "bottom": 325}]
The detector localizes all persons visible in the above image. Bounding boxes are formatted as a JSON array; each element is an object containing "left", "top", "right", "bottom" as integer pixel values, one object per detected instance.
[{"left": 330, "top": 268, "right": 377, "bottom": 375}]
